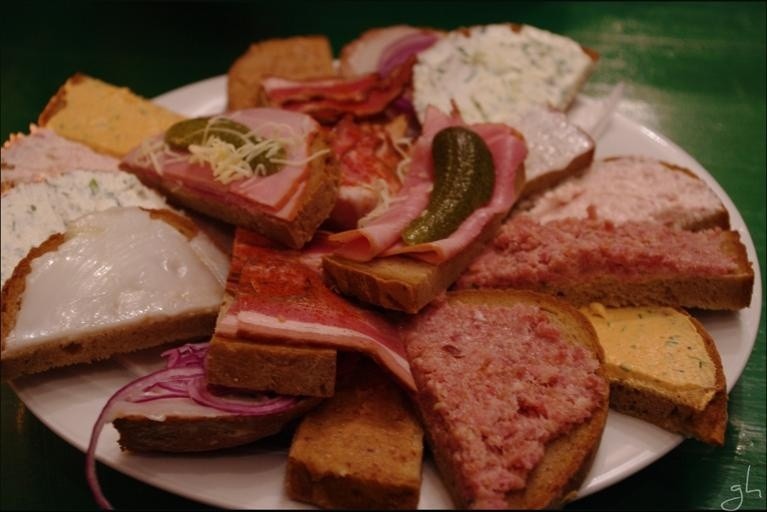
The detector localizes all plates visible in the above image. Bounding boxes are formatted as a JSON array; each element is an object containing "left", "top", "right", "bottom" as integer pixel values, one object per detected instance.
[{"left": 0, "top": 57, "right": 762, "bottom": 509}]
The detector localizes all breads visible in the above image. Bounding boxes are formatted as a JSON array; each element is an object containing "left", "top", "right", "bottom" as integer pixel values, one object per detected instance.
[{"left": 0, "top": 21, "right": 755, "bottom": 512}]
